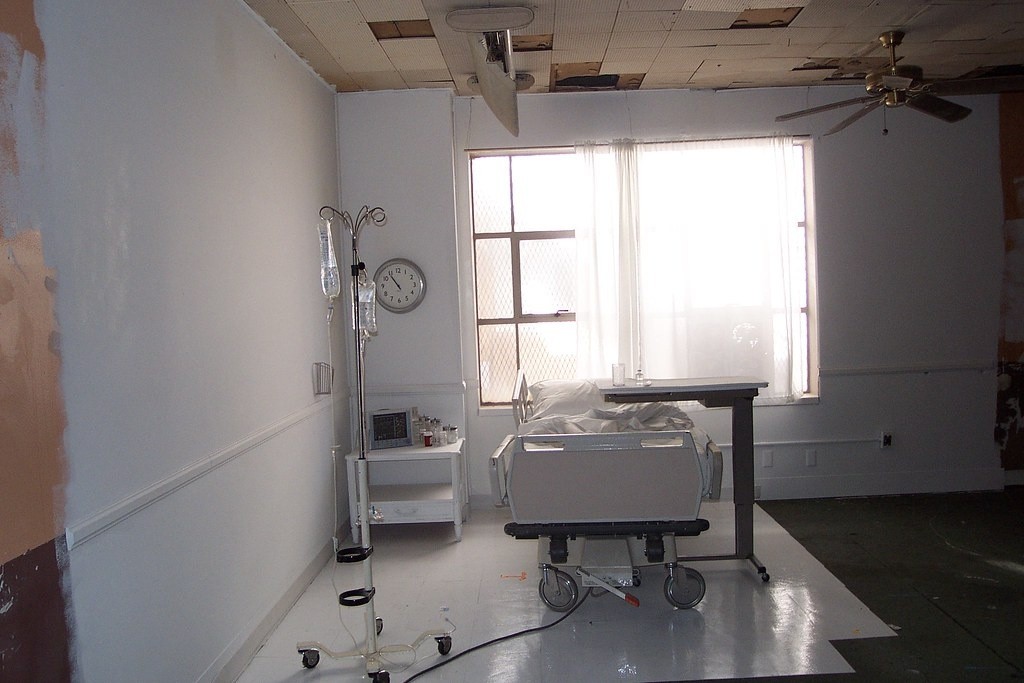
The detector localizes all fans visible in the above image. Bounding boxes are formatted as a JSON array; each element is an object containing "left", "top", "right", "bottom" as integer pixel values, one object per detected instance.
[{"left": 775, "top": 31, "right": 1023, "bottom": 135}]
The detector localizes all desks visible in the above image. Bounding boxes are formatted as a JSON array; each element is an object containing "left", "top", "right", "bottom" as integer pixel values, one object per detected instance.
[{"left": 595, "top": 376, "right": 770, "bottom": 582}]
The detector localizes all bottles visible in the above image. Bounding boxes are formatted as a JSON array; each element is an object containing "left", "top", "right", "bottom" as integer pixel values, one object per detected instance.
[
  {"left": 636, "top": 369, "right": 644, "bottom": 385},
  {"left": 411, "top": 405, "right": 458, "bottom": 448}
]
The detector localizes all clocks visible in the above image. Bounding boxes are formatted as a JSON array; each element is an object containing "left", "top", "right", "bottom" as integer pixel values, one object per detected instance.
[{"left": 373, "top": 258, "right": 427, "bottom": 314}]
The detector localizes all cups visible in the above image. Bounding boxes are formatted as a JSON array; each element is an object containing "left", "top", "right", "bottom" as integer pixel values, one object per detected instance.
[{"left": 611, "top": 362, "right": 625, "bottom": 385}]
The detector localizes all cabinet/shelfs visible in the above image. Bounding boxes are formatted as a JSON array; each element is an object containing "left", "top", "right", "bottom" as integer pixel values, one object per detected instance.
[{"left": 345, "top": 438, "right": 466, "bottom": 544}]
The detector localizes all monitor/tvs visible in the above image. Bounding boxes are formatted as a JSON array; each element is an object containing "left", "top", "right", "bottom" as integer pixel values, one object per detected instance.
[{"left": 367, "top": 407, "right": 413, "bottom": 450}]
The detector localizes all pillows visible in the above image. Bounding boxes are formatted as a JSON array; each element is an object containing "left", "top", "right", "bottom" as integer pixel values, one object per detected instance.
[{"left": 528, "top": 375, "right": 624, "bottom": 418}]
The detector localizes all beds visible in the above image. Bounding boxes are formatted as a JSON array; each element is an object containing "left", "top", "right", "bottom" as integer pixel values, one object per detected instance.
[{"left": 490, "top": 369, "right": 724, "bottom": 611}]
